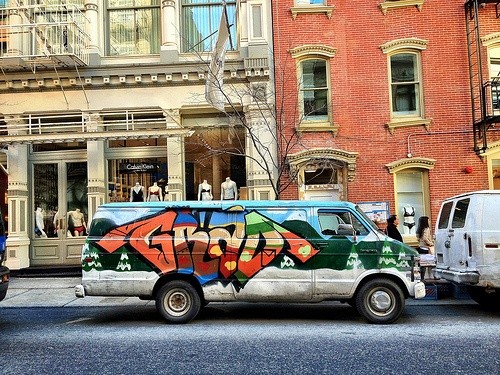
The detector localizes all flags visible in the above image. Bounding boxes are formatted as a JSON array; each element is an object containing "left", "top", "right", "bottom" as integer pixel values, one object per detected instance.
[{"left": 204, "top": 5, "right": 233, "bottom": 119}]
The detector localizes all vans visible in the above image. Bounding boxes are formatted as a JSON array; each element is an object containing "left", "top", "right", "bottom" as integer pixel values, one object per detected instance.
[
  {"left": 432, "top": 190, "right": 500, "bottom": 309},
  {"left": 74, "top": 200, "right": 424, "bottom": 324}
]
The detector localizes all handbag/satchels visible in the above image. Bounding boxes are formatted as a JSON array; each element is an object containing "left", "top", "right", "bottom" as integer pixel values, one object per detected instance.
[{"left": 418, "top": 253, "right": 436, "bottom": 268}]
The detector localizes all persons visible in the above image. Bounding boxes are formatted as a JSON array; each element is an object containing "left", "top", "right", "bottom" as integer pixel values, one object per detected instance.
[
  {"left": 146, "top": 181, "right": 164, "bottom": 202},
  {"left": 129, "top": 181, "right": 147, "bottom": 202},
  {"left": 35, "top": 206, "right": 48, "bottom": 238},
  {"left": 385, "top": 214, "right": 404, "bottom": 245},
  {"left": 416, "top": 215, "right": 435, "bottom": 284},
  {"left": 401, "top": 204, "right": 416, "bottom": 235},
  {"left": 163, "top": 182, "right": 170, "bottom": 201},
  {"left": 197, "top": 179, "right": 214, "bottom": 202},
  {"left": 68, "top": 208, "right": 87, "bottom": 237},
  {"left": 417, "top": 216, "right": 435, "bottom": 280},
  {"left": 52, "top": 208, "right": 73, "bottom": 237},
  {"left": 220, "top": 176, "right": 238, "bottom": 200}
]
